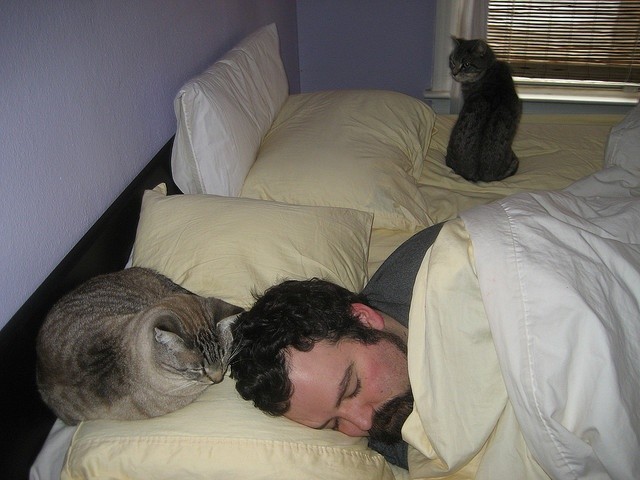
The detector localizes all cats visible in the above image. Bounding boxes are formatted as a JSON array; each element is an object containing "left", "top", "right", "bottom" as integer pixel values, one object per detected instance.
[
  {"left": 445, "top": 34, "right": 522, "bottom": 182},
  {"left": 35, "top": 266, "right": 246, "bottom": 426}
]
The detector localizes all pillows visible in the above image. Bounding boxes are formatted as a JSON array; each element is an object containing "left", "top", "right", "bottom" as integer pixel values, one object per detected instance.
[
  {"left": 170, "top": 22, "right": 291, "bottom": 196},
  {"left": 239, "top": 88, "right": 437, "bottom": 231},
  {"left": 58, "top": 180, "right": 379, "bottom": 480}
]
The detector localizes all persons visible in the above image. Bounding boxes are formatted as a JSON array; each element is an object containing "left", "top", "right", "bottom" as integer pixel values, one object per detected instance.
[{"left": 227, "top": 218, "right": 446, "bottom": 471}]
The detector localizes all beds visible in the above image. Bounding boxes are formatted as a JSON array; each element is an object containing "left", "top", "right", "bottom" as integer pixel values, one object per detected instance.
[{"left": 1, "top": 93, "right": 639, "bottom": 479}]
